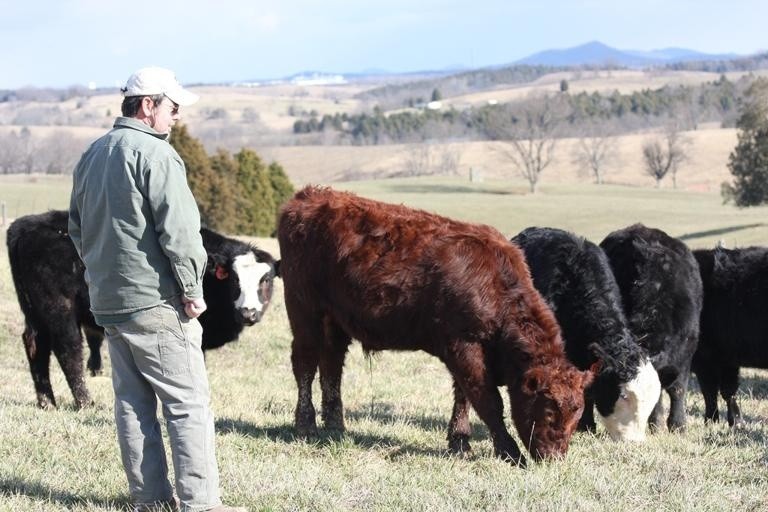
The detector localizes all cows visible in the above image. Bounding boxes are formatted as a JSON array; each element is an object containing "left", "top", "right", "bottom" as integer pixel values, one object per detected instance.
[
  {"left": 275, "top": 180, "right": 596, "bottom": 471},
  {"left": 509, "top": 224, "right": 682, "bottom": 443},
  {"left": 692, "top": 239, "right": 768, "bottom": 429},
  {"left": 5, "top": 208, "right": 284, "bottom": 415},
  {"left": 598, "top": 221, "right": 704, "bottom": 436}
]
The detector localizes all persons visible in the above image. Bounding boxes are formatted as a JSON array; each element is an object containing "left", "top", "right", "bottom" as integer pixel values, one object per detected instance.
[{"left": 68, "top": 68, "right": 248, "bottom": 512}]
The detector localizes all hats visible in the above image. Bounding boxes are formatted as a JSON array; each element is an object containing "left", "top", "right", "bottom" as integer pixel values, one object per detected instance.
[{"left": 124, "top": 67, "right": 199, "bottom": 107}]
